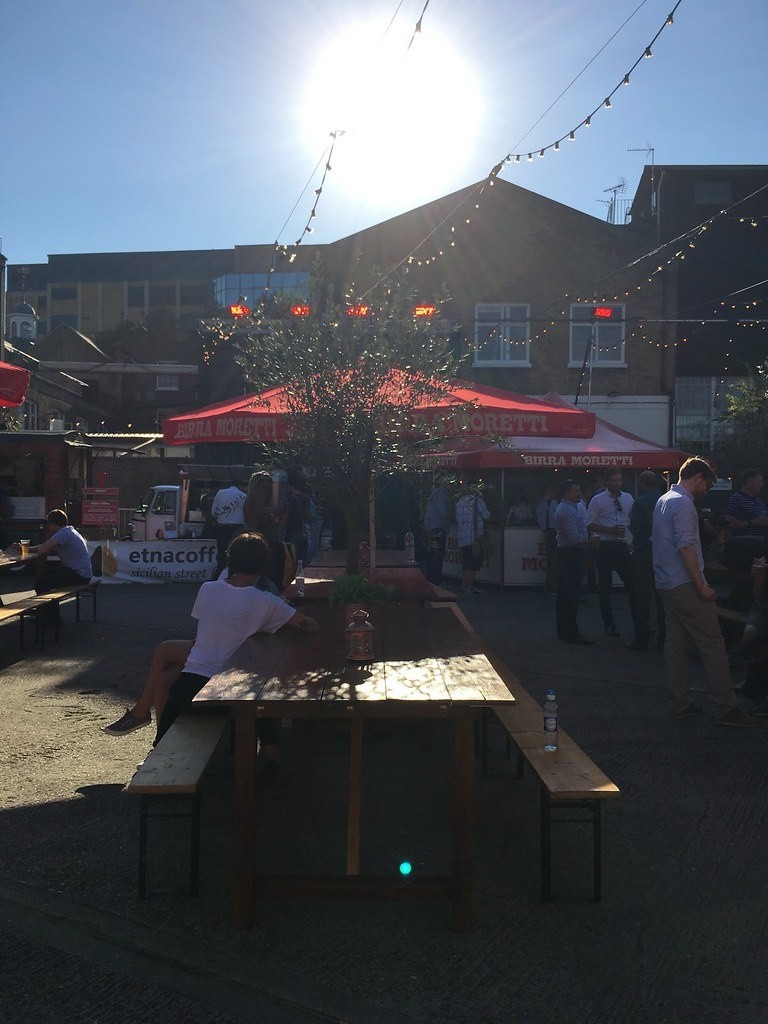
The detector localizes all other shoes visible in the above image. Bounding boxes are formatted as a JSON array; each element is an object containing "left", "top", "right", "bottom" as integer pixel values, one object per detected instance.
[{"left": 557, "top": 633, "right": 585, "bottom": 645}]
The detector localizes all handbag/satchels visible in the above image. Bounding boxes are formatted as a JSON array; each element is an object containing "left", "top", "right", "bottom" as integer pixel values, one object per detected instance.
[{"left": 479, "top": 533, "right": 495, "bottom": 560}]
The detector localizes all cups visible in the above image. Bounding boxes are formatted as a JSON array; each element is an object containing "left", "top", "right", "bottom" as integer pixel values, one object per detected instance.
[{"left": 20, "top": 539, "right": 30, "bottom": 556}]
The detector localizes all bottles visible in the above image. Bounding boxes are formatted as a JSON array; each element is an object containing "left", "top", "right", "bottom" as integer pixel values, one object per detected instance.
[
  {"left": 404, "top": 527, "right": 415, "bottom": 563},
  {"left": 294, "top": 559, "right": 305, "bottom": 597},
  {"left": 544, "top": 689, "right": 559, "bottom": 752}
]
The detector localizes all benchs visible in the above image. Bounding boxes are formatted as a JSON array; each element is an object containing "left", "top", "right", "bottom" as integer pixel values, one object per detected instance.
[
  {"left": 0, "top": 577, "right": 102, "bottom": 651},
  {"left": 427, "top": 580, "right": 458, "bottom": 598},
  {"left": 470, "top": 651, "right": 623, "bottom": 903},
  {"left": 432, "top": 600, "right": 488, "bottom": 663},
  {"left": 121, "top": 714, "right": 228, "bottom": 895}
]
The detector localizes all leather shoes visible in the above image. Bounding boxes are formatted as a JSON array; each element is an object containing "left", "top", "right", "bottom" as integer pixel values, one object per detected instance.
[
  {"left": 649, "top": 628, "right": 656, "bottom": 633},
  {"left": 625, "top": 639, "right": 648, "bottom": 651},
  {"left": 607, "top": 630, "right": 619, "bottom": 636},
  {"left": 657, "top": 642, "right": 664, "bottom": 648}
]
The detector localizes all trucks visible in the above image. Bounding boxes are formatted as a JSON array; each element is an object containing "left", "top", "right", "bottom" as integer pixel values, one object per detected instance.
[{"left": 119, "top": 462, "right": 300, "bottom": 543}]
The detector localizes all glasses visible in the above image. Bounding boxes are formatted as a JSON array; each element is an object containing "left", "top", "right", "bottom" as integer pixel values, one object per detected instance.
[{"left": 614, "top": 499, "right": 623, "bottom": 512}]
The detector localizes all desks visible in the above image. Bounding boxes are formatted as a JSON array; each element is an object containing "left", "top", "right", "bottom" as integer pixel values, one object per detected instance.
[
  {"left": 309, "top": 549, "right": 417, "bottom": 567},
  {"left": 192, "top": 606, "right": 515, "bottom": 875},
  {"left": 277, "top": 566, "right": 440, "bottom": 601}
]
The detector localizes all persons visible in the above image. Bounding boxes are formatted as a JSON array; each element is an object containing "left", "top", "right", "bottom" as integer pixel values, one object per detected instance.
[
  {"left": 554, "top": 480, "right": 595, "bottom": 645},
  {"left": 698, "top": 508, "right": 731, "bottom": 563},
  {"left": 752, "top": 564, "right": 768, "bottom": 717},
  {"left": 423, "top": 479, "right": 454, "bottom": 592},
  {"left": 199, "top": 478, "right": 247, "bottom": 581},
  {"left": 104, "top": 532, "right": 320, "bottom": 760},
  {"left": 243, "top": 470, "right": 321, "bottom": 569},
  {"left": 455, "top": 481, "right": 492, "bottom": 594},
  {"left": 535, "top": 482, "right": 561, "bottom": 595},
  {"left": 575, "top": 486, "right": 599, "bottom": 607},
  {"left": 505, "top": 486, "right": 534, "bottom": 527},
  {"left": 587, "top": 466, "right": 657, "bottom": 637},
  {"left": 21, "top": 509, "right": 93, "bottom": 612},
  {"left": 724, "top": 467, "right": 768, "bottom": 545},
  {"left": 733, "top": 550, "right": 766, "bottom": 691},
  {"left": 652, "top": 456, "right": 762, "bottom": 728},
  {"left": 624, "top": 471, "right": 678, "bottom": 650}
]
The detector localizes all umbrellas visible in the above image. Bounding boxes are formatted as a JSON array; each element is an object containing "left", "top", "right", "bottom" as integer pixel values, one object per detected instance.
[
  {"left": 160, "top": 364, "right": 596, "bottom": 443},
  {"left": 0, "top": 361, "right": 31, "bottom": 407},
  {"left": 393, "top": 390, "right": 716, "bottom": 471}
]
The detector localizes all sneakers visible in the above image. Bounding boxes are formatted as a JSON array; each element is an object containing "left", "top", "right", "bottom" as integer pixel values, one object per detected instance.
[
  {"left": 751, "top": 701, "right": 768, "bottom": 716},
  {"left": 461, "top": 583, "right": 484, "bottom": 594},
  {"left": 100, "top": 709, "right": 152, "bottom": 737},
  {"left": 674, "top": 703, "right": 703, "bottom": 717},
  {"left": 714, "top": 708, "right": 762, "bottom": 728},
  {"left": 733, "top": 678, "right": 747, "bottom": 693}
]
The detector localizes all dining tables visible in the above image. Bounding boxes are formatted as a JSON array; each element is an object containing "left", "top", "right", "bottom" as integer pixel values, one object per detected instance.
[{"left": 0, "top": 548, "right": 45, "bottom": 589}]
